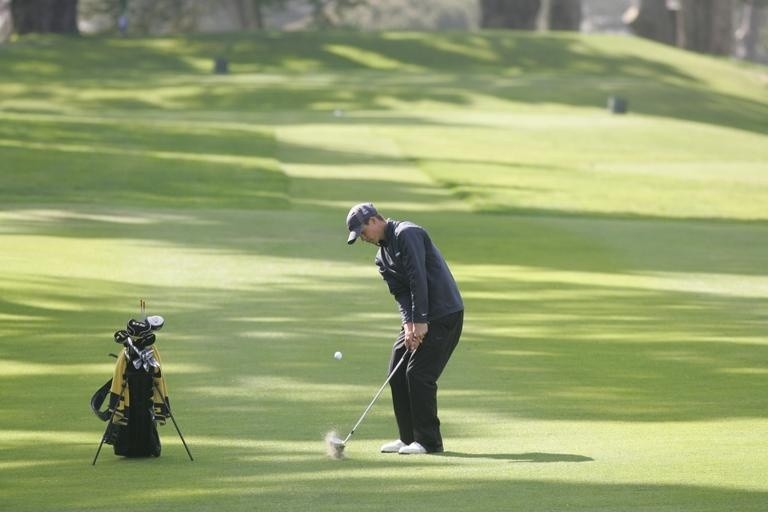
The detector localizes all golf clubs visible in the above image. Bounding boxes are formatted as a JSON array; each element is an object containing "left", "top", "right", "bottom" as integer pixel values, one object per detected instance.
[
  {"left": 330, "top": 346, "right": 411, "bottom": 449},
  {"left": 92, "top": 316, "right": 193, "bottom": 465}
]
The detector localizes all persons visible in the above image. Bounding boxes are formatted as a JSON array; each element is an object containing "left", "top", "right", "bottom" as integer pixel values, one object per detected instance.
[{"left": 344, "top": 202, "right": 465, "bottom": 456}]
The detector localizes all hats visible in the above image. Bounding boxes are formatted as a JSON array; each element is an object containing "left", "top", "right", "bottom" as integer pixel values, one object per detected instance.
[{"left": 347, "top": 201, "right": 376, "bottom": 245}]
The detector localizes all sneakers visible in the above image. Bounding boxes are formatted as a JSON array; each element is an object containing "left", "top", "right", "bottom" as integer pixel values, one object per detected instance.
[
  {"left": 398, "top": 439, "right": 444, "bottom": 455},
  {"left": 380, "top": 440, "right": 405, "bottom": 454}
]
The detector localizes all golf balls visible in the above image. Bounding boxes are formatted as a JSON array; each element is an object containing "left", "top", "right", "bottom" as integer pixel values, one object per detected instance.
[{"left": 335, "top": 351, "right": 342, "bottom": 359}]
